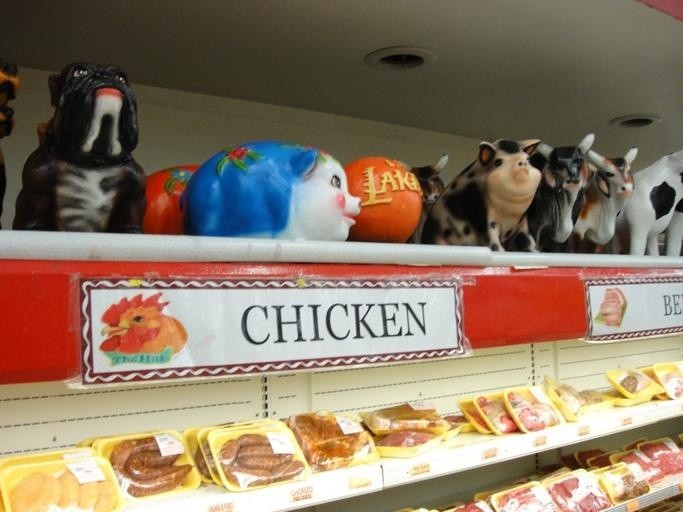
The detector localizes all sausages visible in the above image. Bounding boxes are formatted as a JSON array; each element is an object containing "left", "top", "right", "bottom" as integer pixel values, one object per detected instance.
[{"left": 111, "top": 434, "right": 305, "bottom": 496}]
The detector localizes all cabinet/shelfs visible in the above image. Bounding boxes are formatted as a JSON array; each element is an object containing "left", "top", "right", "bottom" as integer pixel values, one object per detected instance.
[{"left": 0, "top": 230, "right": 683, "bottom": 512}]
